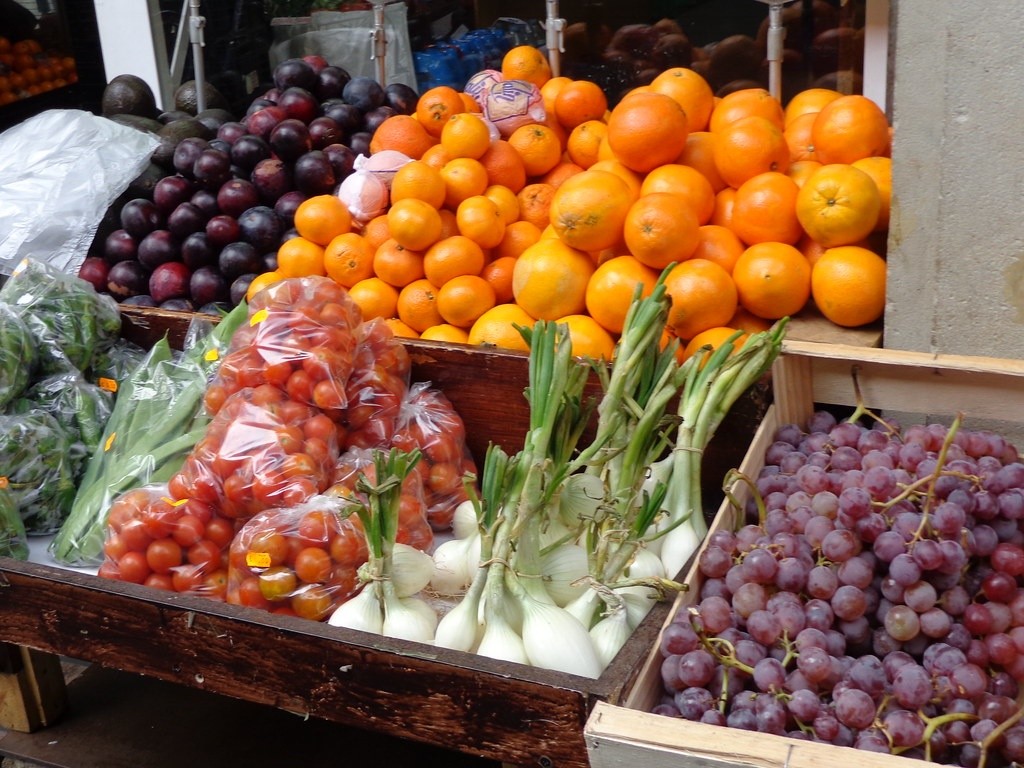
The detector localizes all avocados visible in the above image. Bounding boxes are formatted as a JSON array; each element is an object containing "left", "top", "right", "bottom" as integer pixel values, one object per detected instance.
[{"left": 88, "top": 74, "right": 239, "bottom": 256}]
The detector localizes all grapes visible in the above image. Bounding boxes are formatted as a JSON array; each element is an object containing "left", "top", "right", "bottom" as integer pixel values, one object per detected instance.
[{"left": 651, "top": 411, "right": 1024, "bottom": 768}]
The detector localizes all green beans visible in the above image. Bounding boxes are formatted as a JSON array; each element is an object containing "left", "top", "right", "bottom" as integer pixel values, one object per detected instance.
[{"left": 44, "top": 294, "right": 249, "bottom": 567}]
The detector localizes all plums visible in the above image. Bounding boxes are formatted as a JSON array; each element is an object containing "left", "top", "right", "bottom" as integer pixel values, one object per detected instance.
[{"left": 76, "top": 53, "right": 420, "bottom": 315}]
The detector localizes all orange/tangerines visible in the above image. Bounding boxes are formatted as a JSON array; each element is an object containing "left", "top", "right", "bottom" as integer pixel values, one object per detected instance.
[
  {"left": 1, "top": 36, "right": 78, "bottom": 106},
  {"left": 245, "top": 44, "right": 894, "bottom": 372},
  {"left": 538, "top": 1, "right": 865, "bottom": 111}
]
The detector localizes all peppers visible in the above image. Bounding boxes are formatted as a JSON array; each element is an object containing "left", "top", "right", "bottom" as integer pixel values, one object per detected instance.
[{"left": 1, "top": 256, "right": 121, "bottom": 561}]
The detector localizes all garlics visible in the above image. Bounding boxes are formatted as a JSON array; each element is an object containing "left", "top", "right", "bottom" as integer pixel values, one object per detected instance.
[{"left": 326, "top": 265, "right": 791, "bottom": 679}]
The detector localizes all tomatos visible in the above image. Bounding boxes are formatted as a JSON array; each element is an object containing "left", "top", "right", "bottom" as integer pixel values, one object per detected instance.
[{"left": 99, "top": 275, "right": 478, "bottom": 623}]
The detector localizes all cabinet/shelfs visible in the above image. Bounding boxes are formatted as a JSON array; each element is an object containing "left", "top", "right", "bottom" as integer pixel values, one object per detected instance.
[{"left": 0, "top": 0, "right": 1023, "bottom": 768}]
